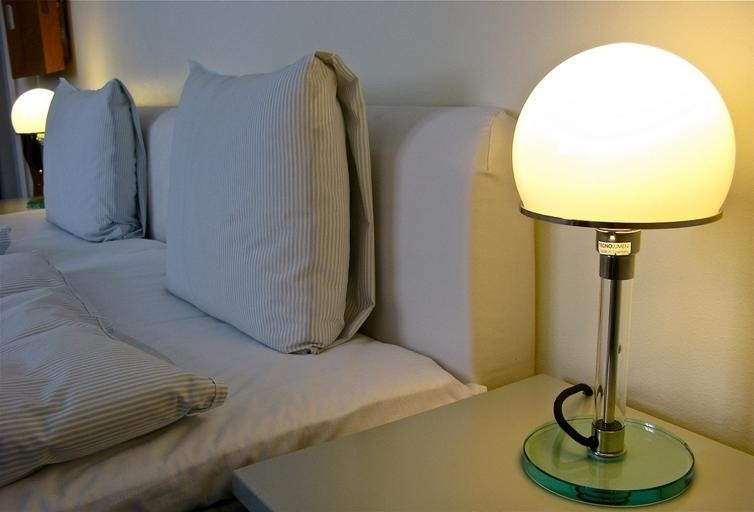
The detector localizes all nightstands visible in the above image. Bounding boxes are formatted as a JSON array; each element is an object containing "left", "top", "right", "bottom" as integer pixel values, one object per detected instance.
[
  {"left": 232, "top": 372, "right": 753, "bottom": 512},
  {"left": 0, "top": 195, "right": 43, "bottom": 215}
]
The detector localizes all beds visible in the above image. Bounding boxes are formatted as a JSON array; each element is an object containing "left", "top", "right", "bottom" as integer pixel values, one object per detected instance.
[{"left": 0, "top": 102, "right": 539, "bottom": 512}]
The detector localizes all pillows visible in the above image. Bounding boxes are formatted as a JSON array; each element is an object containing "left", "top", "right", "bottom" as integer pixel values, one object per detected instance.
[
  {"left": 161, "top": 51, "right": 379, "bottom": 355},
  {"left": 43, "top": 76, "right": 148, "bottom": 244}
]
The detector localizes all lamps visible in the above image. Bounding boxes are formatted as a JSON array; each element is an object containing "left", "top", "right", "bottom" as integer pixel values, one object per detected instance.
[
  {"left": 511, "top": 40, "right": 734, "bottom": 508},
  {"left": 11, "top": 86, "right": 57, "bottom": 205}
]
